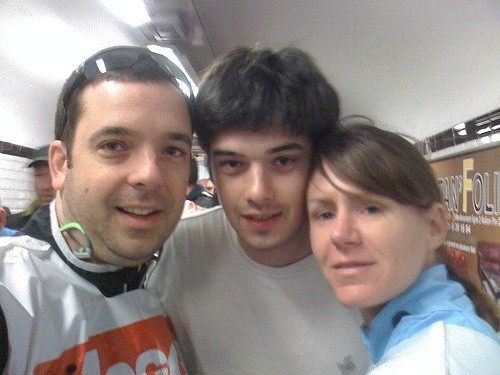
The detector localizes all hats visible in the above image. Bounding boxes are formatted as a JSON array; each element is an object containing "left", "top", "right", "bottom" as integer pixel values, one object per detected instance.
[{"left": 27, "top": 143, "right": 54, "bottom": 171}]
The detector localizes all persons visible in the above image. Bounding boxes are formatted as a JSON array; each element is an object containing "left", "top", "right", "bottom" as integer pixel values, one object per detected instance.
[
  {"left": 0, "top": 47, "right": 192, "bottom": 375},
  {"left": 305, "top": 112, "right": 499, "bottom": 375},
  {"left": 185, "top": 153, "right": 218, "bottom": 208},
  {"left": 7, "top": 145, "right": 56, "bottom": 229},
  {"left": 140, "top": 39, "right": 374, "bottom": 375}
]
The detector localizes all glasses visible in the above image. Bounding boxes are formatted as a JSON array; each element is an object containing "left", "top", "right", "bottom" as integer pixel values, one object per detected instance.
[{"left": 61, "top": 44, "right": 200, "bottom": 114}]
